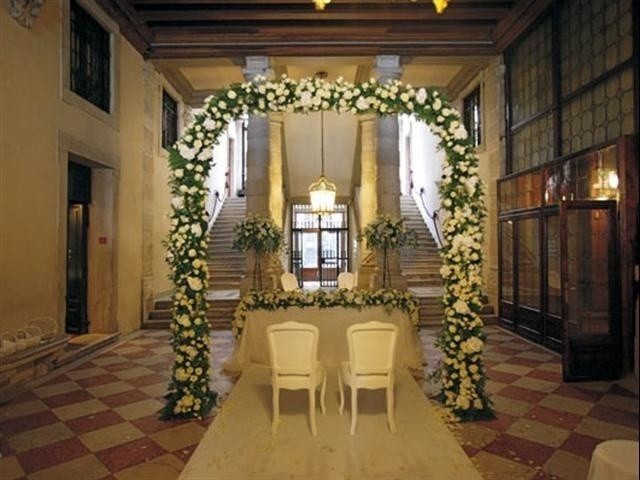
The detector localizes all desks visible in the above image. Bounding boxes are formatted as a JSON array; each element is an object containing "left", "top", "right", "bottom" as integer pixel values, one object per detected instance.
[{"left": 230, "top": 287, "right": 423, "bottom": 370}]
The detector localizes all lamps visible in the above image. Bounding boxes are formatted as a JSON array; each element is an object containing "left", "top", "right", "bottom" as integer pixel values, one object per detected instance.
[{"left": 307, "top": 72, "right": 337, "bottom": 213}]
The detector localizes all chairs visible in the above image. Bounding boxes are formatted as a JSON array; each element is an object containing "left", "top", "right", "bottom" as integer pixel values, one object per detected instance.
[
  {"left": 267, "top": 319, "right": 328, "bottom": 434},
  {"left": 336, "top": 320, "right": 401, "bottom": 437}
]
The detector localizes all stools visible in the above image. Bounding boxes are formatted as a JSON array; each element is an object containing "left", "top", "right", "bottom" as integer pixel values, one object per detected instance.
[{"left": 585, "top": 441, "right": 639, "bottom": 479}]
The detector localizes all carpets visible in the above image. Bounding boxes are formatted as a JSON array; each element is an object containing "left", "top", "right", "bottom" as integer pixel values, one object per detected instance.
[{"left": 174, "top": 369, "right": 485, "bottom": 480}]
